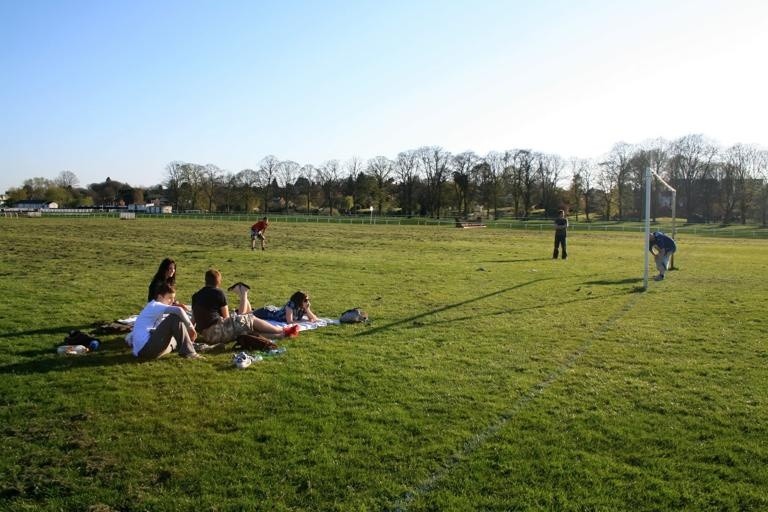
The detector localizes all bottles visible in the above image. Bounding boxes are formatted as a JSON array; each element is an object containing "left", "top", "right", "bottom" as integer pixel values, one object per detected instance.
[
  {"left": 88, "top": 339, "right": 102, "bottom": 352},
  {"left": 249, "top": 338, "right": 286, "bottom": 361},
  {"left": 56, "top": 344, "right": 91, "bottom": 358}
]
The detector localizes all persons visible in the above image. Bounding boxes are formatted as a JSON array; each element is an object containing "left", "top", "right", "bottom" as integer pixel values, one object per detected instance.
[
  {"left": 250, "top": 216, "right": 269, "bottom": 251},
  {"left": 649, "top": 232, "right": 676, "bottom": 280},
  {"left": 227, "top": 282, "right": 318, "bottom": 324},
  {"left": 190, "top": 269, "right": 300, "bottom": 345},
  {"left": 132, "top": 283, "right": 208, "bottom": 361},
  {"left": 552, "top": 209, "right": 568, "bottom": 259},
  {"left": 148, "top": 257, "right": 190, "bottom": 313}
]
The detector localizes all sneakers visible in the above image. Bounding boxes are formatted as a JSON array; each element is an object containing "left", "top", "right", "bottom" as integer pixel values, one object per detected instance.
[{"left": 233, "top": 352, "right": 263, "bottom": 369}]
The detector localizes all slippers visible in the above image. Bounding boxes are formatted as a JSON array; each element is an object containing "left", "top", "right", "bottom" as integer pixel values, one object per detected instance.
[
  {"left": 228, "top": 282, "right": 242, "bottom": 291},
  {"left": 239, "top": 282, "right": 250, "bottom": 290}
]
